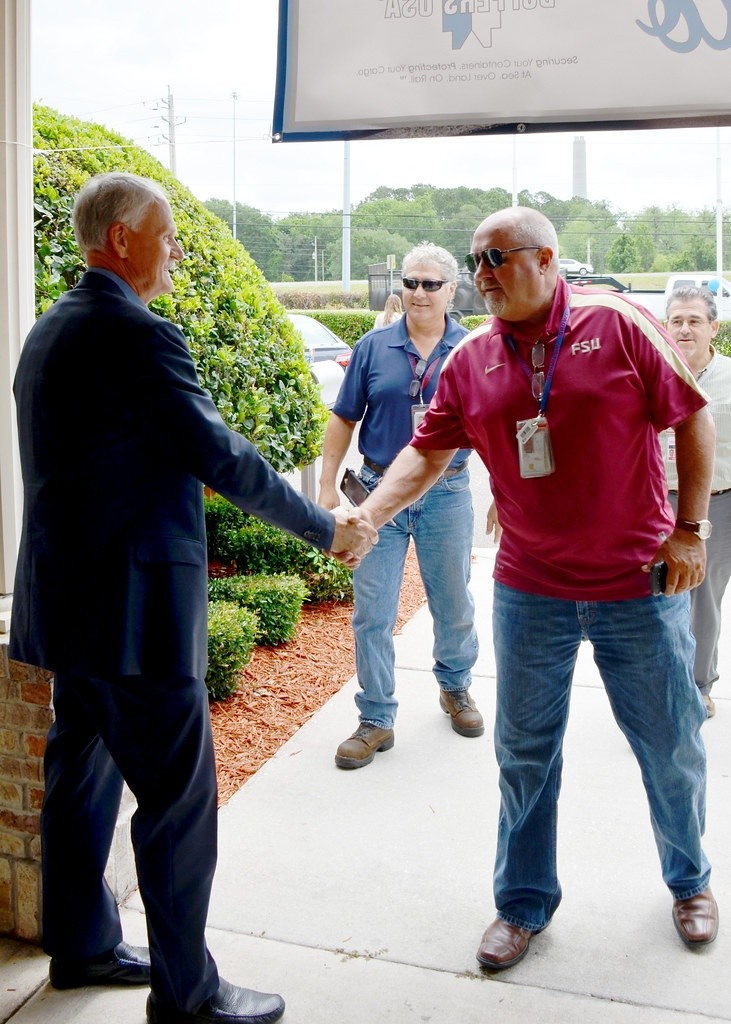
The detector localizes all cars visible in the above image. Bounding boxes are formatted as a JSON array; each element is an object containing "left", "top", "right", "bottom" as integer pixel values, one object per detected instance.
[{"left": 288, "top": 312, "right": 353, "bottom": 412}]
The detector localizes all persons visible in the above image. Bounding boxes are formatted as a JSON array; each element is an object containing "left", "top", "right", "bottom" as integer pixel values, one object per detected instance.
[
  {"left": 375, "top": 294, "right": 402, "bottom": 330},
  {"left": 322, "top": 206, "right": 718, "bottom": 967},
  {"left": 316, "top": 241, "right": 504, "bottom": 769},
  {"left": 8, "top": 171, "right": 379, "bottom": 1024},
  {"left": 656, "top": 285, "right": 731, "bottom": 718}
]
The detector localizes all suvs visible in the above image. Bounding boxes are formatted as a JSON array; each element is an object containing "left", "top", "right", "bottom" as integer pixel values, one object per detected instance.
[{"left": 559, "top": 259, "right": 593, "bottom": 275}]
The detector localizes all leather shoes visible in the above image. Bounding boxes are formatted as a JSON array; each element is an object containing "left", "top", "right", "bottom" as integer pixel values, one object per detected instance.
[
  {"left": 671, "top": 884, "right": 719, "bottom": 947},
  {"left": 147, "top": 976, "right": 285, "bottom": 1024},
  {"left": 476, "top": 914, "right": 552, "bottom": 969},
  {"left": 49, "top": 942, "right": 152, "bottom": 990}
]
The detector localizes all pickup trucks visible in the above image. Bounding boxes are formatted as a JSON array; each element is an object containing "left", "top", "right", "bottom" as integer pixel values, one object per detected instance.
[{"left": 622, "top": 273, "right": 731, "bottom": 322}]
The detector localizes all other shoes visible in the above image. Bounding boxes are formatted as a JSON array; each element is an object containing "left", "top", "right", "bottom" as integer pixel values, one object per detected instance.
[{"left": 701, "top": 694, "right": 715, "bottom": 717}]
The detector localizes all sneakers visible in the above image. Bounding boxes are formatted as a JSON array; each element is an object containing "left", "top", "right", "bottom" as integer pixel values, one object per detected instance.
[
  {"left": 440, "top": 686, "right": 484, "bottom": 738},
  {"left": 335, "top": 722, "right": 395, "bottom": 767}
]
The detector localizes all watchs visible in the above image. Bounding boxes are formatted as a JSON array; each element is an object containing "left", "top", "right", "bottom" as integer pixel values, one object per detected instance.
[{"left": 675, "top": 519, "right": 713, "bottom": 540}]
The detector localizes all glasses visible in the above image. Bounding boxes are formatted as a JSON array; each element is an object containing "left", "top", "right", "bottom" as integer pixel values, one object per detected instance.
[
  {"left": 464, "top": 246, "right": 542, "bottom": 273},
  {"left": 401, "top": 277, "right": 449, "bottom": 293}
]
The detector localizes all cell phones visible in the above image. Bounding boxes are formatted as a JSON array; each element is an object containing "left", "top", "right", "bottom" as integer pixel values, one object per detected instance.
[{"left": 651, "top": 562, "right": 668, "bottom": 596}]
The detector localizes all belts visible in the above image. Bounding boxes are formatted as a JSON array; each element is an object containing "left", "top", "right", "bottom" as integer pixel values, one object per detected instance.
[
  {"left": 667, "top": 488, "right": 731, "bottom": 496},
  {"left": 363, "top": 456, "right": 467, "bottom": 481}
]
